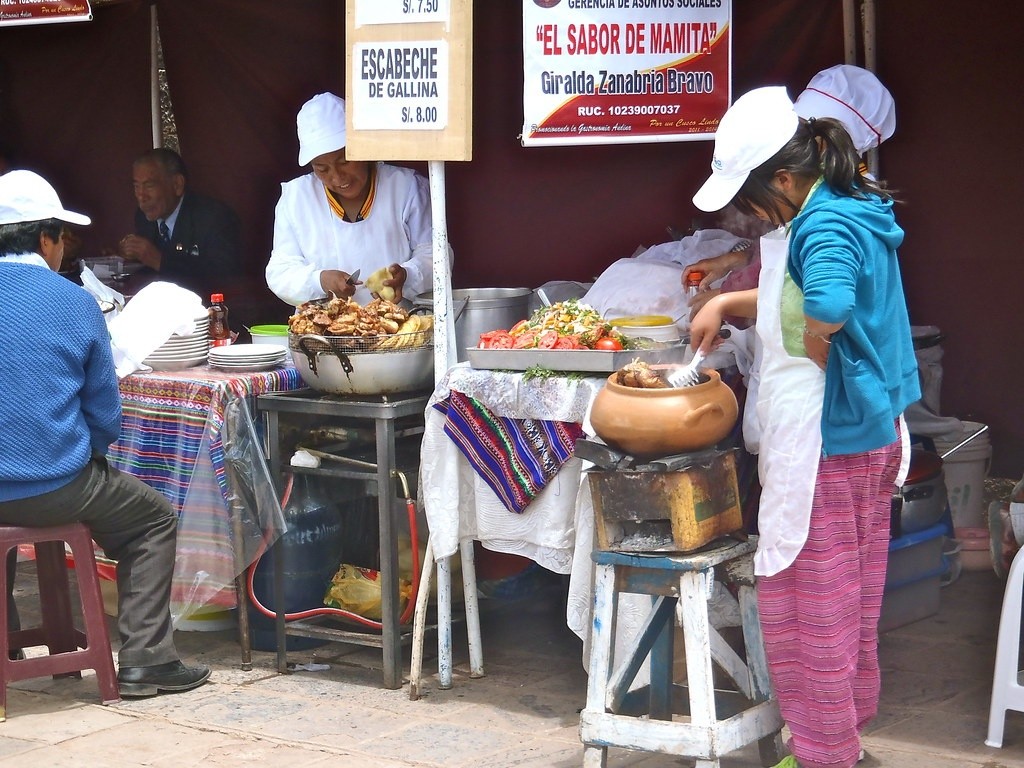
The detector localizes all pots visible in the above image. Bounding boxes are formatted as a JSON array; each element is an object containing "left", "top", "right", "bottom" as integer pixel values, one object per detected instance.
[
  {"left": 286, "top": 305, "right": 442, "bottom": 397},
  {"left": 888, "top": 449, "right": 948, "bottom": 536}
]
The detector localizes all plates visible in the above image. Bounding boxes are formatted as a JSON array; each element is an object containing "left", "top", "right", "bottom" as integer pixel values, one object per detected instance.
[{"left": 137, "top": 307, "right": 290, "bottom": 373}]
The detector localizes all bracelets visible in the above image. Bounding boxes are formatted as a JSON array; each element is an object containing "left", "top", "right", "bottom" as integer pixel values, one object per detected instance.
[{"left": 806, "top": 324, "right": 820, "bottom": 339}]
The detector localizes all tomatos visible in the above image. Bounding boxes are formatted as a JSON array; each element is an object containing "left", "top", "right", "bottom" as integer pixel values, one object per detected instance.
[{"left": 478, "top": 321, "right": 624, "bottom": 351}]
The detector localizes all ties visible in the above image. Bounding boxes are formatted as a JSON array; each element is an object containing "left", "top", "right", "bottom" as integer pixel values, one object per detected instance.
[{"left": 160, "top": 221, "right": 170, "bottom": 246}]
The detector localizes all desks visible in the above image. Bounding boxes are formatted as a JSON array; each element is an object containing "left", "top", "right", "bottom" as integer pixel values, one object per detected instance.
[
  {"left": 421, "top": 361, "right": 739, "bottom": 696},
  {"left": 15, "top": 362, "right": 312, "bottom": 672}
]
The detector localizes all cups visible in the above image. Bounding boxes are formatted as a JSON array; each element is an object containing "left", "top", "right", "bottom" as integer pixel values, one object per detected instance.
[
  {"left": 687, "top": 273, "right": 713, "bottom": 303},
  {"left": 208, "top": 294, "right": 232, "bottom": 353}
]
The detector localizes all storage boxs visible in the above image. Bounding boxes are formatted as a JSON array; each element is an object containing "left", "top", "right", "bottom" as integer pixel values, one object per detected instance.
[{"left": 875, "top": 523, "right": 952, "bottom": 634}]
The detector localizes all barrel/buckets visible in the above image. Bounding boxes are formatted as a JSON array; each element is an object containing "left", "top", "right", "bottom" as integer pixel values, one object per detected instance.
[
  {"left": 616, "top": 323, "right": 682, "bottom": 342},
  {"left": 393, "top": 472, "right": 464, "bottom": 605},
  {"left": 414, "top": 285, "right": 535, "bottom": 365},
  {"left": 248, "top": 323, "right": 292, "bottom": 359},
  {"left": 921, "top": 416, "right": 995, "bottom": 531}
]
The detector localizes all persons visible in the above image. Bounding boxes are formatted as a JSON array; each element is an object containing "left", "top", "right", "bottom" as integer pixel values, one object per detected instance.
[
  {"left": 681, "top": 64, "right": 896, "bottom": 330},
  {"left": 691, "top": 86, "right": 922, "bottom": 768},
  {"left": 265, "top": 92, "right": 453, "bottom": 308},
  {"left": 0, "top": 170, "right": 213, "bottom": 694},
  {"left": 61, "top": 149, "right": 240, "bottom": 305}
]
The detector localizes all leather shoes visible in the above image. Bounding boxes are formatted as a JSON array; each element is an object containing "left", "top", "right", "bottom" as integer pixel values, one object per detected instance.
[{"left": 117, "top": 660, "right": 212, "bottom": 697}]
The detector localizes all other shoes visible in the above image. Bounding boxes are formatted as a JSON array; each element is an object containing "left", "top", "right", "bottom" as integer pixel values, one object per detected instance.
[{"left": 771, "top": 754, "right": 802, "bottom": 768}]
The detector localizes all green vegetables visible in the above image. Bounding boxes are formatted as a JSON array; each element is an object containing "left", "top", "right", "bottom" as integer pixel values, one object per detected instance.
[{"left": 525, "top": 298, "right": 646, "bottom": 351}]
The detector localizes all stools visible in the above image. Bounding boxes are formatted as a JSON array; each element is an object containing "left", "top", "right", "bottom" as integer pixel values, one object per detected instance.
[
  {"left": 985, "top": 543, "right": 1024, "bottom": 748},
  {"left": 575, "top": 536, "right": 786, "bottom": 768},
  {"left": 0, "top": 521, "right": 122, "bottom": 722}
]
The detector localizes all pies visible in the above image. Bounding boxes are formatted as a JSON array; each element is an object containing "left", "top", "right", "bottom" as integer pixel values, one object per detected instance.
[
  {"left": 380, "top": 314, "right": 434, "bottom": 348},
  {"left": 363, "top": 267, "right": 395, "bottom": 302}
]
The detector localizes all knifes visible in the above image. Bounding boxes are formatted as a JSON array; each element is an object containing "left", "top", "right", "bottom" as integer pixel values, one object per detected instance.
[{"left": 346, "top": 269, "right": 360, "bottom": 286}]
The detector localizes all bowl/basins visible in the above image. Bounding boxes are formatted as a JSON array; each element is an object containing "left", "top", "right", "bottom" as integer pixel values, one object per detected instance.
[
  {"left": 81, "top": 255, "right": 138, "bottom": 279},
  {"left": 589, "top": 367, "right": 739, "bottom": 456},
  {"left": 953, "top": 526, "right": 992, "bottom": 572}
]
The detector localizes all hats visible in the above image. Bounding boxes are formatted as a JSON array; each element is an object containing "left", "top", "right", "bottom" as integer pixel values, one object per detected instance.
[
  {"left": 0, "top": 170, "right": 92, "bottom": 226},
  {"left": 692, "top": 85, "right": 799, "bottom": 213},
  {"left": 794, "top": 64, "right": 896, "bottom": 158},
  {"left": 297, "top": 92, "right": 345, "bottom": 167}
]
